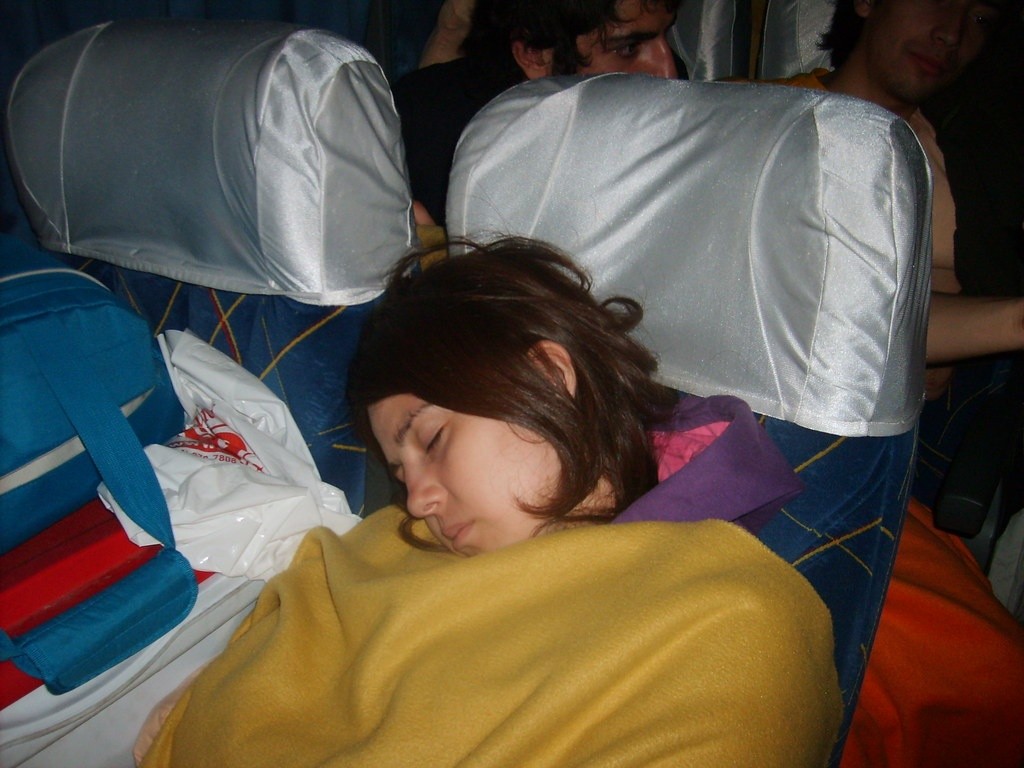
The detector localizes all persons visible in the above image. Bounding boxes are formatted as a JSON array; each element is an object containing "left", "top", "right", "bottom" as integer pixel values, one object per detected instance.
[
  {"left": 709, "top": 0, "right": 1024, "bottom": 577},
  {"left": 393, "top": 0, "right": 680, "bottom": 238},
  {"left": 346, "top": 232, "right": 803, "bottom": 557}
]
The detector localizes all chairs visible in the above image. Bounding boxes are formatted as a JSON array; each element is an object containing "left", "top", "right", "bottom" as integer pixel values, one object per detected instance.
[{"left": 0, "top": 0, "right": 929, "bottom": 767}]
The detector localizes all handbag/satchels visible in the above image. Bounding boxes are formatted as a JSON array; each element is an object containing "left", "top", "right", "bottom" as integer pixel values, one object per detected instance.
[
  {"left": 97, "top": 326, "right": 366, "bottom": 582},
  {"left": 0, "top": 231, "right": 200, "bottom": 697}
]
